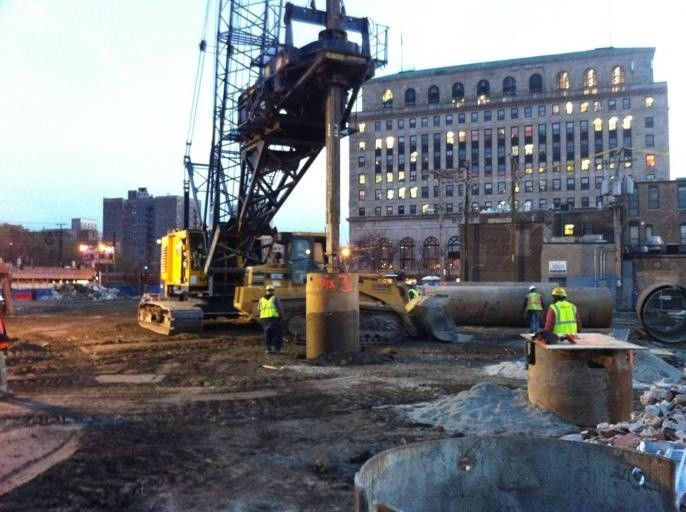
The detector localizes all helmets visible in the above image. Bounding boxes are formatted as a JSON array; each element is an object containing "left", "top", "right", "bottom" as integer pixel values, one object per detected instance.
[
  {"left": 528, "top": 284, "right": 536, "bottom": 291},
  {"left": 552, "top": 287, "right": 568, "bottom": 298},
  {"left": 265, "top": 285, "right": 276, "bottom": 290}
]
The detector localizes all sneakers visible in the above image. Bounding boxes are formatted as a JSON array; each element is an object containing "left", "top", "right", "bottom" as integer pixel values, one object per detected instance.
[{"left": 264, "top": 349, "right": 283, "bottom": 355}]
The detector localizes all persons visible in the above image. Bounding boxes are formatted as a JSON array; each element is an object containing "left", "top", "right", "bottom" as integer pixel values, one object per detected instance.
[
  {"left": 288, "top": 242, "right": 308, "bottom": 274},
  {"left": 268, "top": 251, "right": 284, "bottom": 280},
  {"left": 191, "top": 243, "right": 202, "bottom": 270},
  {"left": 255, "top": 285, "right": 287, "bottom": 356},
  {"left": 544, "top": 285, "right": 582, "bottom": 336},
  {"left": 406, "top": 284, "right": 419, "bottom": 303},
  {"left": 396, "top": 268, "right": 408, "bottom": 282},
  {"left": 0, "top": 295, "right": 15, "bottom": 401},
  {"left": 519, "top": 284, "right": 544, "bottom": 334}
]
